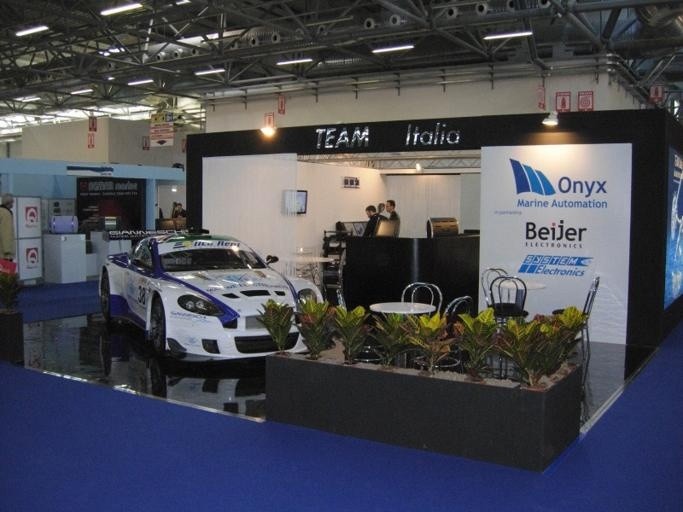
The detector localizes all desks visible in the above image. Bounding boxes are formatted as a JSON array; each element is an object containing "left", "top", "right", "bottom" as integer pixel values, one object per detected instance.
[{"left": 283, "top": 256, "right": 334, "bottom": 302}]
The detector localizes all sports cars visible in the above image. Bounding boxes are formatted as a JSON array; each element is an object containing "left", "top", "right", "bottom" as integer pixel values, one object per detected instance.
[{"left": 98, "top": 233, "right": 326, "bottom": 364}]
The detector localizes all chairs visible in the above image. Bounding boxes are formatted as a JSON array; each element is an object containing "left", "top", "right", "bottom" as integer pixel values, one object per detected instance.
[
  {"left": 427, "top": 217, "right": 459, "bottom": 238},
  {"left": 351, "top": 268, "right": 600, "bottom": 425}
]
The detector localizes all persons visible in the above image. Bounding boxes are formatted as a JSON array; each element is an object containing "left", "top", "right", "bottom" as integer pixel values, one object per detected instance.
[
  {"left": 362, "top": 205, "right": 387, "bottom": 237},
  {"left": 387, "top": 200, "right": 400, "bottom": 237},
  {"left": 175, "top": 203, "right": 187, "bottom": 217},
  {"left": 155, "top": 203, "right": 163, "bottom": 218},
  {"left": 0, "top": 193, "right": 16, "bottom": 262},
  {"left": 172, "top": 202, "right": 177, "bottom": 218}
]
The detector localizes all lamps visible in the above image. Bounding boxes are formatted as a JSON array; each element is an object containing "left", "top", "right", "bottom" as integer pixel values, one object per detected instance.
[
  {"left": 261, "top": 128, "right": 276, "bottom": 137},
  {"left": 542, "top": 111, "right": 559, "bottom": 127}
]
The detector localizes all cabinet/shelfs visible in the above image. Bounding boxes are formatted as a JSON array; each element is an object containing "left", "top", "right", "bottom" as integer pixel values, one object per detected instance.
[{"left": 42, "top": 234, "right": 87, "bottom": 284}]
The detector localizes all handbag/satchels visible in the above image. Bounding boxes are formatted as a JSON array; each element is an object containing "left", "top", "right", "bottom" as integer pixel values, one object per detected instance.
[{"left": 0, "top": 259, "right": 16, "bottom": 274}]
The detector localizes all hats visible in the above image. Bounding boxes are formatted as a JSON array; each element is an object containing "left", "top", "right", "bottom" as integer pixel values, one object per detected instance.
[{"left": 2, "top": 195, "right": 14, "bottom": 205}]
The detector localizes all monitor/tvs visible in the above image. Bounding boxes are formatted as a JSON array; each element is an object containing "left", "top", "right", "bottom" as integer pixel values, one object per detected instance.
[{"left": 281, "top": 189, "right": 307, "bottom": 214}]
